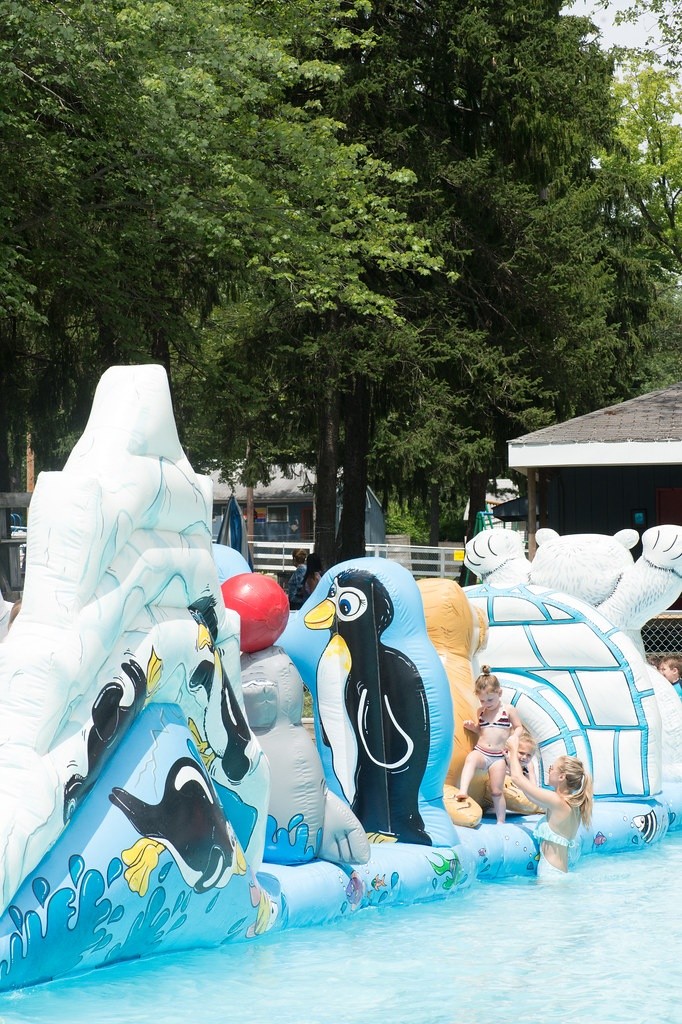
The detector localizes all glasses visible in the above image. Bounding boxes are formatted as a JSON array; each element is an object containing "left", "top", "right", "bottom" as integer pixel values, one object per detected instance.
[{"left": 549, "top": 765, "right": 561, "bottom": 774}]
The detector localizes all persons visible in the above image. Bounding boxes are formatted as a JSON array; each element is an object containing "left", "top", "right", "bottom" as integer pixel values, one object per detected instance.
[
  {"left": 517, "top": 731, "right": 536, "bottom": 786},
  {"left": 648, "top": 656, "right": 664, "bottom": 670},
  {"left": 500, "top": 735, "right": 592, "bottom": 876},
  {"left": 8, "top": 599, "right": 22, "bottom": 632},
  {"left": 287, "top": 549, "right": 307, "bottom": 610},
  {"left": 302, "top": 554, "right": 323, "bottom": 600},
  {"left": 455, "top": 664, "right": 522, "bottom": 824},
  {"left": 658, "top": 656, "right": 682, "bottom": 701}
]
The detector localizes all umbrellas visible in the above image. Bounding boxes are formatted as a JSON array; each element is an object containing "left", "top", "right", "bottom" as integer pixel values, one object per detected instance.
[{"left": 218, "top": 494, "right": 254, "bottom": 573}]
